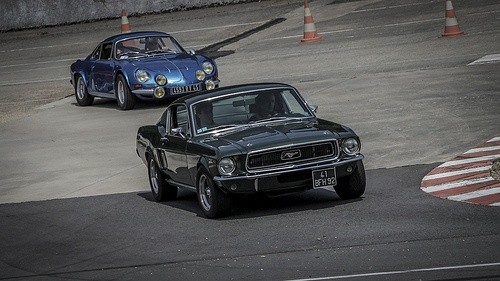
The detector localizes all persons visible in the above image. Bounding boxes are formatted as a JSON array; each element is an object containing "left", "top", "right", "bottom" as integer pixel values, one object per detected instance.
[
  {"left": 248, "top": 92, "right": 280, "bottom": 123},
  {"left": 195, "top": 102, "right": 218, "bottom": 132},
  {"left": 146, "top": 37, "right": 169, "bottom": 52},
  {"left": 116, "top": 43, "right": 125, "bottom": 59}
]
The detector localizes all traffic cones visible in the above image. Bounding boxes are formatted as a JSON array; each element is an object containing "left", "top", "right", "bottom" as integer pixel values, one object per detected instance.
[
  {"left": 440, "top": 0, "right": 465, "bottom": 38},
  {"left": 121, "top": 8, "right": 131, "bottom": 32},
  {"left": 300, "top": 0, "right": 322, "bottom": 43}
]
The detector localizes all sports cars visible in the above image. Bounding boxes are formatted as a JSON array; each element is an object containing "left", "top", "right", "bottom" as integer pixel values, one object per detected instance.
[
  {"left": 135, "top": 81, "right": 367, "bottom": 216},
  {"left": 68, "top": 30, "right": 219, "bottom": 109}
]
